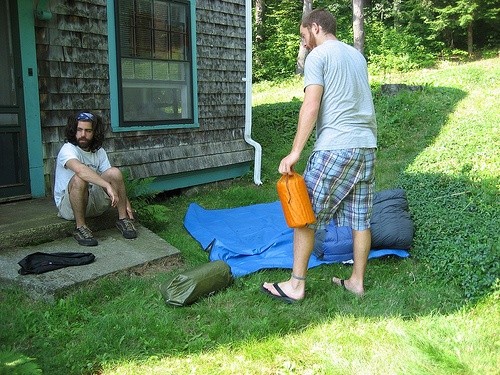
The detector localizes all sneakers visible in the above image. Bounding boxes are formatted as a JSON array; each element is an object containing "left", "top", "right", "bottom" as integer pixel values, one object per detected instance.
[
  {"left": 115, "top": 217, "right": 137, "bottom": 239},
  {"left": 72, "top": 225, "right": 98, "bottom": 246}
]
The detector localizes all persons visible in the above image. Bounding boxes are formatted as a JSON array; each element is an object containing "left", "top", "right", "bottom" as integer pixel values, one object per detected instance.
[
  {"left": 261, "top": 8, "right": 376, "bottom": 301},
  {"left": 53, "top": 111, "right": 137, "bottom": 246}
]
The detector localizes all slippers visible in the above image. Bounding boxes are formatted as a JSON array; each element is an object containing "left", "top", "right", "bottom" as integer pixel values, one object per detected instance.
[
  {"left": 260, "top": 282, "right": 297, "bottom": 302},
  {"left": 331, "top": 276, "right": 349, "bottom": 293}
]
